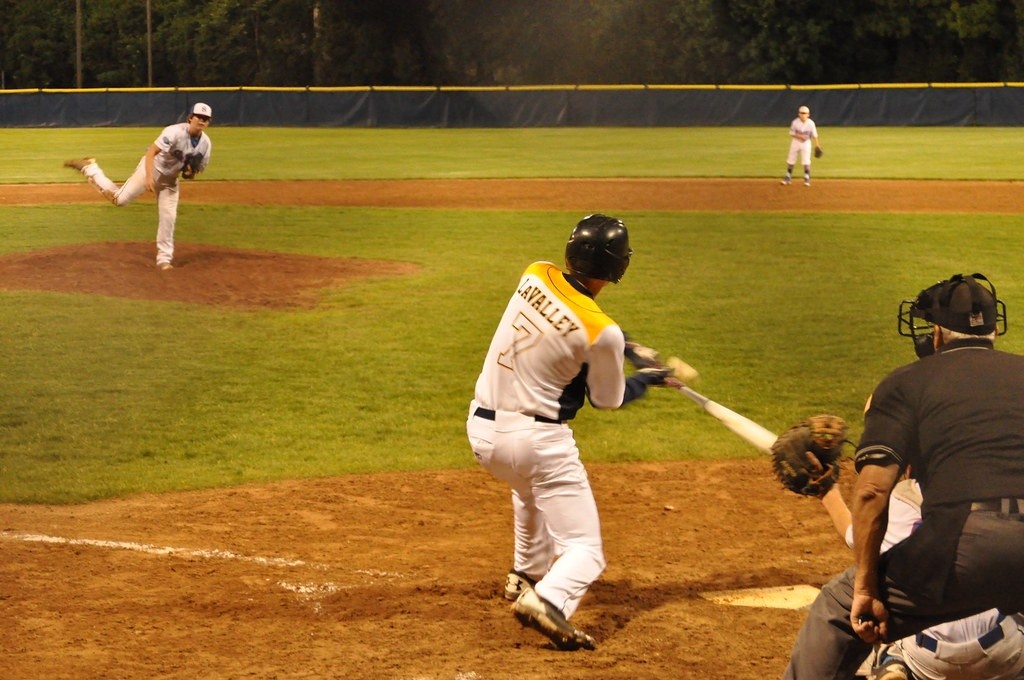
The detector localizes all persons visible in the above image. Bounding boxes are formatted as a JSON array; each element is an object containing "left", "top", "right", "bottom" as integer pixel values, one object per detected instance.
[
  {"left": 63, "top": 103, "right": 213, "bottom": 270},
  {"left": 466, "top": 211, "right": 682, "bottom": 649},
  {"left": 778, "top": 106, "right": 822, "bottom": 187},
  {"left": 770, "top": 271, "right": 1023, "bottom": 680}
]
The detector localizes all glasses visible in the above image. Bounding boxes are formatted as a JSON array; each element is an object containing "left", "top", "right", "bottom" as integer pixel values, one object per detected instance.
[{"left": 194, "top": 114, "right": 210, "bottom": 121}]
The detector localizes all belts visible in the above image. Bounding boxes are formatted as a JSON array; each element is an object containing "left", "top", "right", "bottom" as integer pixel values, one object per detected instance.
[
  {"left": 474, "top": 407, "right": 564, "bottom": 423},
  {"left": 915, "top": 625, "right": 1005, "bottom": 652},
  {"left": 971, "top": 496, "right": 1019, "bottom": 513}
]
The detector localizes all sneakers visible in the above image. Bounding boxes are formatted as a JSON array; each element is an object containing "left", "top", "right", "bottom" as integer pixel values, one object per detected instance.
[
  {"left": 64, "top": 157, "right": 95, "bottom": 170},
  {"left": 161, "top": 263, "right": 173, "bottom": 270},
  {"left": 505, "top": 569, "right": 538, "bottom": 599},
  {"left": 512, "top": 588, "right": 596, "bottom": 649}
]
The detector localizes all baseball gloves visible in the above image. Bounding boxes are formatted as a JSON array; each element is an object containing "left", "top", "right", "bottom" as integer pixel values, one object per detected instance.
[
  {"left": 770, "top": 414, "right": 848, "bottom": 499},
  {"left": 814, "top": 146, "right": 824, "bottom": 158},
  {"left": 181, "top": 151, "right": 204, "bottom": 180}
]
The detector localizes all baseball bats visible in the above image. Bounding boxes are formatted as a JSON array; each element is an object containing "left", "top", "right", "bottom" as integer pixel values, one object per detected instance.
[{"left": 662, "top": 374, "right": 778, "bottom": 455}]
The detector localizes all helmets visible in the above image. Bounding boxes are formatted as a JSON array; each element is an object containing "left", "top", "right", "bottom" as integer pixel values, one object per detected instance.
[{"left": 566, "top": 214, "right": 632, "bottom": 283}]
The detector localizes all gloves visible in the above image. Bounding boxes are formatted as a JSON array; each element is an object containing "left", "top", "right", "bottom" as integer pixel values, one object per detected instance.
[
  {"left": 624, "top": 341, "right": 663, "bottom": 369},
  {"left": 634, "top": 367, "right": 674, "bottom": 387}
]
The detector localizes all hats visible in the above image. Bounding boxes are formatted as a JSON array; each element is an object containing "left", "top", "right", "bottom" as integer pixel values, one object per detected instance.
[
  {"left": 910, "top": 279, "right": 997, "bottom": 335},
  {"left": 799, "top": 106, "right": 809, "bottom": 113},
  {"left": 192, "top": 103, "right": 212, "bottom": 117}
]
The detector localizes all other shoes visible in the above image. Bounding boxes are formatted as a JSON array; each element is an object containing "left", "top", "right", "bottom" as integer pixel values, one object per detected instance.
[
  {"left": 804, "top": 183, "right": 809, "bottom": 186},
  {"left": 780, "top": 180, "right": 792, "bottom": 185},
  {"left": 877, "top": 660, "right": 909, "bottom": 680}
]
